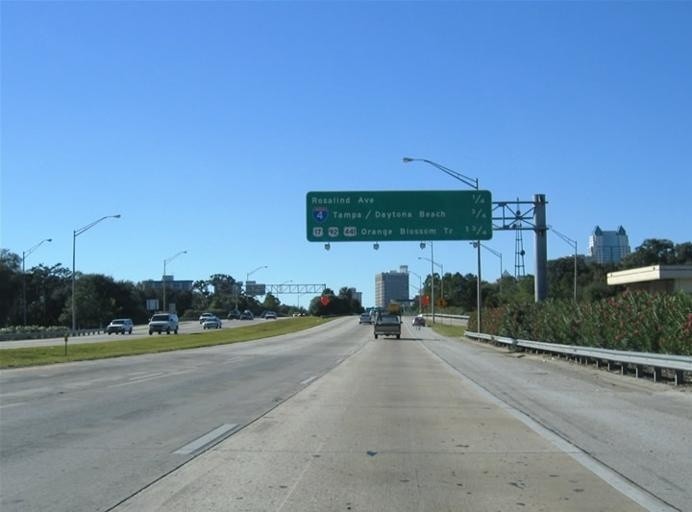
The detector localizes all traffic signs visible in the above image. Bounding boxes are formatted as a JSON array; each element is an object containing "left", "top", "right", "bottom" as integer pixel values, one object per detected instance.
[{"left": 303, "top": 188, "right": 495, "bottom": 245}]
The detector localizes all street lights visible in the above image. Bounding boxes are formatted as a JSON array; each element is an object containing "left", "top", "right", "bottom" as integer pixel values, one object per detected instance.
[
  {"left": 405, "top": 254, "right": 446, "bottom": 315},
  {"left": 400, "top": 156, "right": 483, "bottom": 346},
  {"left": 245, "top": 265, "right": 269, "bottom": 281},
  {"left": 277, "top": 279, "right": 293, "bottom": 298},
  {"left": 21, "top": 238, "right": 53, "bottom": 327},
  {"left": 71, "top": 213, "right": 123, "bottom": 331},
  {"left": 161, "top": 250, "right": 187, "bottom": 312}
]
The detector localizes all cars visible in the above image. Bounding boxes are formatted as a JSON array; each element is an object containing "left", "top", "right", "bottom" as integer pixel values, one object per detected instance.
[
  {"left": 358, "top": 309, "right": 404, "bottom": 341},
  {"left": 197, "top": 308, "right": 278, "bottom": 331},
  {"left": 410, "top": 316, "right": 426, "bottom": 326}
]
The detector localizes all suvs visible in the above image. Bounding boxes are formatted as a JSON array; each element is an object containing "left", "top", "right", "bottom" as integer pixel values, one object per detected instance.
[{"left": 105, "top": 318, "right": 133, "bottom": 336}]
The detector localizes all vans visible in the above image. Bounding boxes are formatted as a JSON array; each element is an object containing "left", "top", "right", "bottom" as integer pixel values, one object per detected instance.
[{"left": 147, "top": 312, "right": 179, "bottom": 336}]
